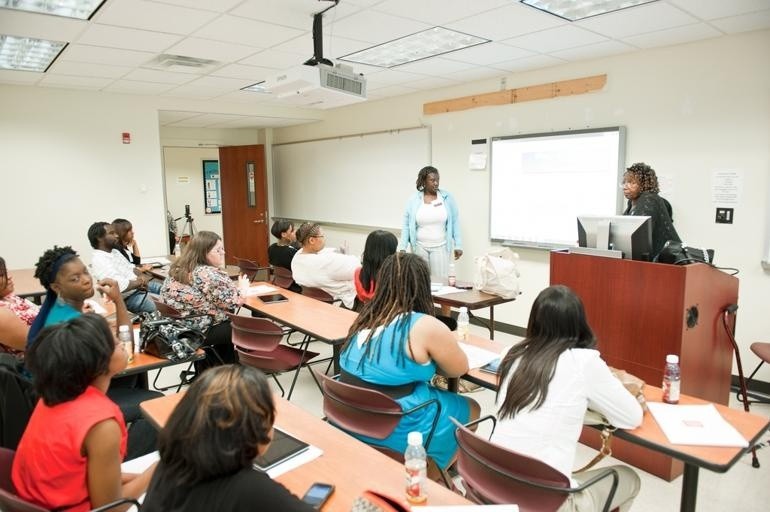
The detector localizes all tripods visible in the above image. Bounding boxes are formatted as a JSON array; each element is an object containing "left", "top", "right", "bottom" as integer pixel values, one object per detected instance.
[{"left": 172, "top": 215, "right": 197, "bottom": 254}]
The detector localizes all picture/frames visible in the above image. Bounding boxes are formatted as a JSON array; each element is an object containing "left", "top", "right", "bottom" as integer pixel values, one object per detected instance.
[{"left": 203, "top": 160, "right": 221, "bottom": 214}]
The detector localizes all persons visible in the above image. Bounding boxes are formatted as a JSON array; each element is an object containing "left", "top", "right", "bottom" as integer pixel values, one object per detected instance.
[
  {"left": 111, "top": 218, "right": 141, "bottom": 266},
  {"left": 354, "top": 229, "right": 398, "bottom": 306},
  {"left": 325, "top": 248, "right": 473, "bottom": 496},
  {"left": 621, "top": 162, "right": 683, "bottom": 264},
  {"left": 17, "top": 243, "right": 135, "bottom": 375},
  {"left": 138, "top": 363, "right": 325, "bottom": 511},
  {"left": 88, "top": 222, "right": 164, "bottom": 319},
  {"left": 267, "top": 219, "right": 303, "bottom": 294},
  {"left": 159, "top": 230, "right": 240, "bottom": 377},
  {"left": 291, "top": 221, "right": 365, "bottom": 313},
  {"left": 167, "top": 210, "right": 180, "bottom": 255},
  {"left": 396, "top": 165, "right": 465, "bottom": 281},
  {"left": 485, "top": 284, "right": 644, "bottom": 512},
  {"left": 0, "top": 256, "right": 39, "bottom": 359},
  {"left": 8, "top": 310, "right": 163, "bottom": 511}
]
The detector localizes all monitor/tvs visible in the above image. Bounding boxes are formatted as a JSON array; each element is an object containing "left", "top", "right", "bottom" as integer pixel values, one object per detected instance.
[{"left": 576, "top": 215, "right": 654, "bottom": 262}]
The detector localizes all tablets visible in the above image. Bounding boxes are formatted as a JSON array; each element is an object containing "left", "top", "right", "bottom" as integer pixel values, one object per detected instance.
[
  {"left": 257, "top": 294, "right": 288, "bottom": 304},
  {"left": 253, "top": 428, "right": 309, "bottom": 472}
]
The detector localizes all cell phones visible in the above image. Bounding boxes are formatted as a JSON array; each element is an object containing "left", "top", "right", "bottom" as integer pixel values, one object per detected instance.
[{"left": 301, "top": 482, "right": 334, "bottom": 510}]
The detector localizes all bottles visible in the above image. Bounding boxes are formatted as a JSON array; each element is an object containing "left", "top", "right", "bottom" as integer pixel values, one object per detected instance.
[
  {"left": 402, "top": 432, "right": 428, "bottom": 505},
  {"left": 118, "top": 324, "right": 135, "bottom": 365},
  {"left": 662, "top": 354, "right": 681, "bottom": 404},
  {"left": 174, "top": 243, "right": 182, "bottom": 258},
  {"left": 448, "top": 267, "right": 456, "bottom": 287}
]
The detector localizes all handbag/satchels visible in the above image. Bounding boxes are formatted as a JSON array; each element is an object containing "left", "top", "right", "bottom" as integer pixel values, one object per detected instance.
[
  {"left": 657, "top": 242, "right": 715, "bottom": 266},
  {"left": 140, "top": 310, "right": 215, "bottom": 363},
  {"left": 475, "top": 247, "right": 521, "bottom": 299},
  {"left": 352, "top": 489, "right": 412, "bottom": 511},
  {"left": 584, "top": 367, "right": 646, "bottom": 425}
]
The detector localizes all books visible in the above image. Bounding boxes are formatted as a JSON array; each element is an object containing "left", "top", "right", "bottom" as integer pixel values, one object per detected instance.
[{"left": 480, "top": 358, "right": 502, "bottom": 373}]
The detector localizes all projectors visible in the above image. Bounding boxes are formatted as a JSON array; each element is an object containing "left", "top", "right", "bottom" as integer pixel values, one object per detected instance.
[{"left": 264, "top": 62, "right": 368, "bottom": 110}]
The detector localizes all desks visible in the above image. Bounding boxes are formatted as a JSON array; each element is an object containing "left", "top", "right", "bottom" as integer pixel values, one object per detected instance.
[{"left": 448, "top": 330, "right": 770, "bottom": 512}]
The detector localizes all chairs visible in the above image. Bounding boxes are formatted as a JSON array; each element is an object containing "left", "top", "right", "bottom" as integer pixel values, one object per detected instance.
[{"left": 737, "top": 342, "right": 770, "bottom": 403}]
